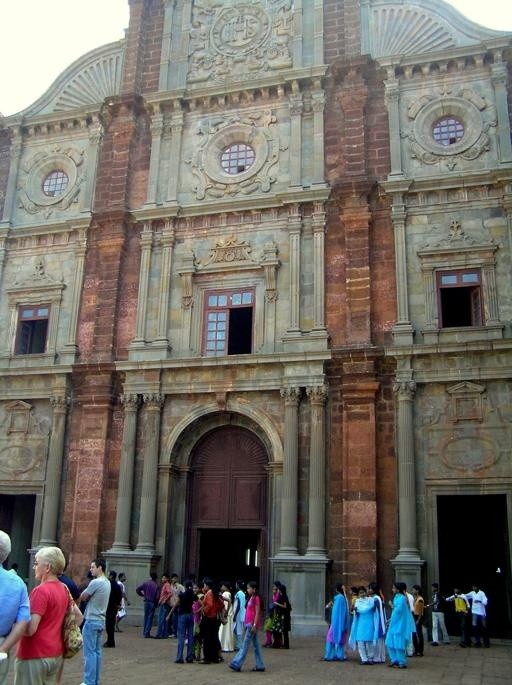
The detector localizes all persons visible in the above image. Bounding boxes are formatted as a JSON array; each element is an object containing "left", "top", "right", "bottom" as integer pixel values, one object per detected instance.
[
  {"left": 80, "top": 571, "right": 131, "bottom": 648},
  {"left": 9, "top": 561, "right": 18, "bottom": 574},
  {"left": 423, "top": 583, "right": 451, "bottom": 646},
  {"left": 77, "top": 558, "right": 112, "bottom": 685},
  {"left": 136, "top": 572, "right": 266, "bottom": 671},
  {"left": 1, "top": 531, "right": 33, "bottom": 685},
  {"left": 57, "top": 557, "right": 80, "bottom": 685},
  {"left": 320, "top": 582, "right": 424, "bottom": 669},
  {"left": 464, "top": 583, "right": 488, "bottom": 648},
  {"left": 442, "top": 586, "right": 473, "bottom": 648},
  {"left": 14, "top": 547, "right": 84, "bottom": 685},
  {"left": 260, "top": 581, "right": 292, "bottom": 649}
]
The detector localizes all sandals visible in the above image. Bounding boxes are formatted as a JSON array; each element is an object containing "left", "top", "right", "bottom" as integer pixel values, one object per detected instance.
[
  {"left": 393, "top": 664, "right": 406, "bottom": 668},
  {"left": 252, "top": 666, "right": 265, "bottom": 672},
  {"left": 387, "top": 660, "right": 399, "bottom": 666},
  {"left": 229, "top": 664, "right": 241, "bottom": 671},
  {"left": 219, "top": 656, "right": 224, "bottom": 661}
]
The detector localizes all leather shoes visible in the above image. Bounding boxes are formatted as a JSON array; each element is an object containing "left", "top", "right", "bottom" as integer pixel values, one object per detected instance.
[{"left": 196, "top": 655, "right": 211, "bottom": 664}]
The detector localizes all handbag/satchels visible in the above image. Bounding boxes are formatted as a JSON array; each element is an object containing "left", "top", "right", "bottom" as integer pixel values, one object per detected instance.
[
  {"left": 218, "top": 608, "right": 228, "bottom": 625},
  {"left": 62, "top": 583, "right": 84, "bottom": 659}
]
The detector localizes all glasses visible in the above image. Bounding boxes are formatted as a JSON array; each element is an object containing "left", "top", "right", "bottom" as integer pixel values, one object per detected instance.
[{"left": 33, "top": 562, "right": 39, "bottom": 566}]
